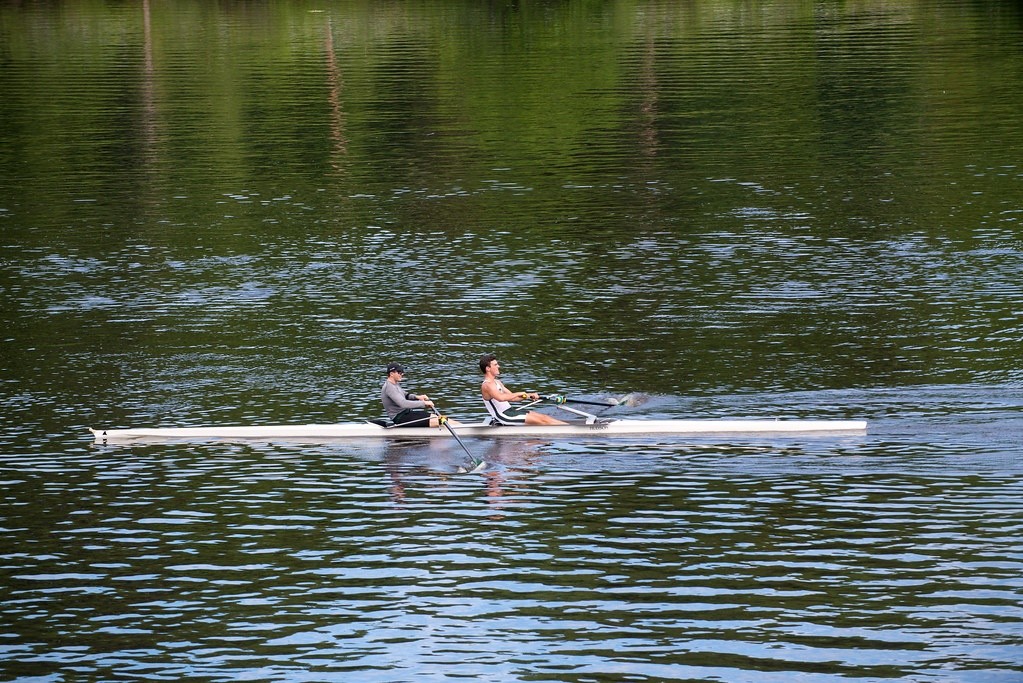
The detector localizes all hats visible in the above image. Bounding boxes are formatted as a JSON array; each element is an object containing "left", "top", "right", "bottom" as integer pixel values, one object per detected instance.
[{"left": 386, "top": 363, "right": 406, "bottom": 372}]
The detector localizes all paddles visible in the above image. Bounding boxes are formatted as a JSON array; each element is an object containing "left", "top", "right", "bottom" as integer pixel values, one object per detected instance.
[
  {"left": 431, "top": 405, "right": 483, "bottom": 472},
  {"left": 523, "top": 393, "right": 628, "bottom": 406}
]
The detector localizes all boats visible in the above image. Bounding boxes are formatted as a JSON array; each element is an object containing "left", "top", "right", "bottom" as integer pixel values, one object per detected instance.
[{"left": 92, "top": 411, "right": 867, "bottom": 434}]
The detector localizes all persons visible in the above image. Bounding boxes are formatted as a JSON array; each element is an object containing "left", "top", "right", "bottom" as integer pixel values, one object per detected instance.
[
  {"left": 480, "top": 356, "right": 569, "bottom": 426},
  {"left": 381, "top": 363, "right": 463, "bottom": 427}
]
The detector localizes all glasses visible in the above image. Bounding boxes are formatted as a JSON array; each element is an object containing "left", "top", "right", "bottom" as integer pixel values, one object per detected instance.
[{"left": 393, "top": 370, "right": 405, "bottom": 374}]
